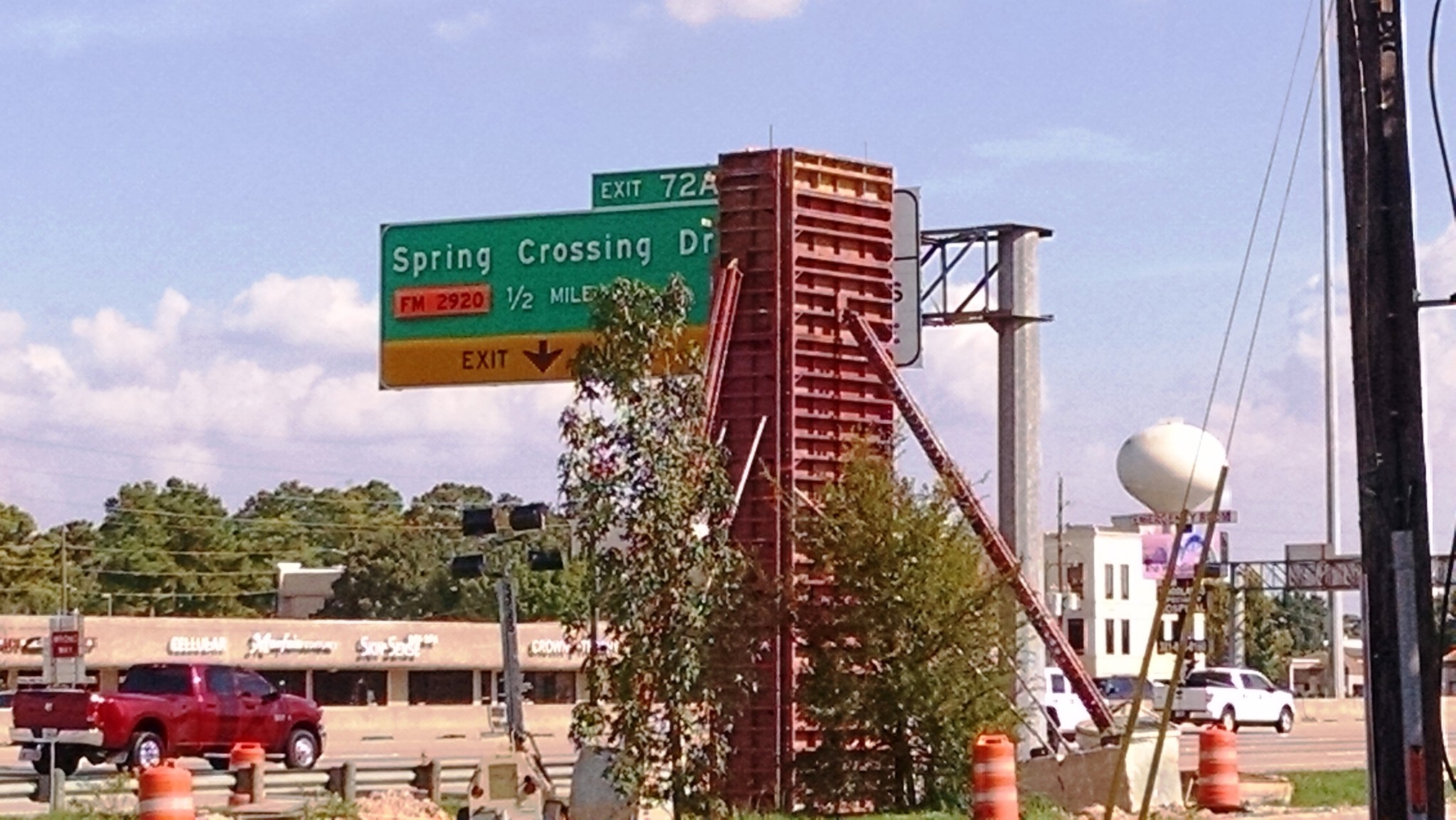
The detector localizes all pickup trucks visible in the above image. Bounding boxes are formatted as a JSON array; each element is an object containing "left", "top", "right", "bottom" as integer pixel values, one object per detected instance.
[
  {"left": 1041, "top": 666, "right": 1111, "bottom": 738},
  {"left": 8, "top": 660, "right": 329, "bottom": 779},
  {"left": 1150, "top": 666, "right": 1298, "bottom": 736}
]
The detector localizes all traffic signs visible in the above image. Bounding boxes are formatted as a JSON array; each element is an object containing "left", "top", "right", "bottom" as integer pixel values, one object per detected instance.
[{"left": 377, "top": 198, "right": 722, "bottom": 391}]
